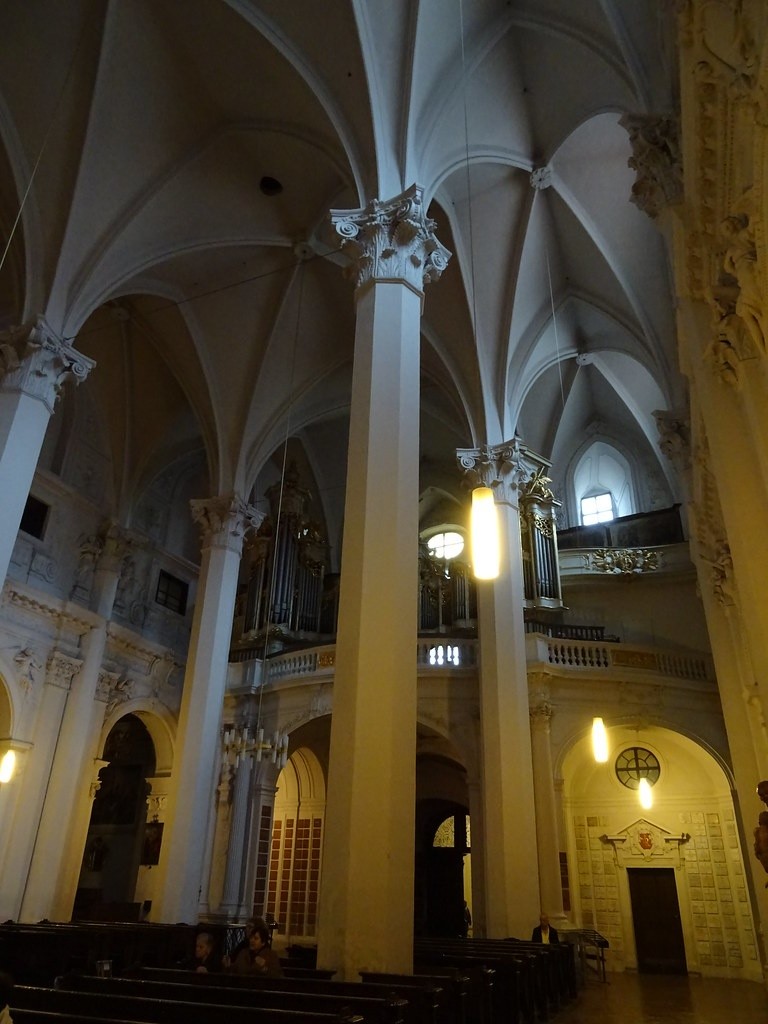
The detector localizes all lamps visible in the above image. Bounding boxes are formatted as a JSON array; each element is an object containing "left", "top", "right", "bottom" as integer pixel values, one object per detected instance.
[{"left": 221, "top": 252, "right": 306, "bottom": 768}]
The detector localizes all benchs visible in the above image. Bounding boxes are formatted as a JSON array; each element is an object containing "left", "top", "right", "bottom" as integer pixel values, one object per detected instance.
[{"left": 0, "top": 919, "right": 578, "bottom": 1024}]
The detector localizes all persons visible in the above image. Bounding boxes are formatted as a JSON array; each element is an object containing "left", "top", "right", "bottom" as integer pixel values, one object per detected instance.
[
  {"left": 224, "top": 916, "right": 283, "bottom": 976},
  {"left": 532, "top": 912, "right": 560, "bottom": 945},
  {"left": 193, "top": 932, "right": 227, "bottom": 974},
  {"left": 719, "top": 216, "right": 767, "bottom": 359},
  {"left": 463, "top": 900, "right": 471, "bottom": 928}
]
